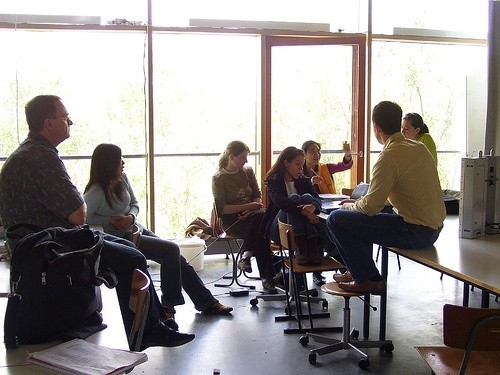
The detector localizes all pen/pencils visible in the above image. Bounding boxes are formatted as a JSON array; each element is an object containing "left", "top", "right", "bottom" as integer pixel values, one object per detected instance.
[{"left": 311, "top": 168, "right": 324, "bottom": 182}]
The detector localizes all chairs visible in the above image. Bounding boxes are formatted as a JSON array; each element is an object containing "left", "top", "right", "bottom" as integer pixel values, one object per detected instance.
[
  {"left": 126, "top": 269, "right": 152, "bottom": 375},
  {"left": 414, "top": 304, "right": 500, "bottom": 375},
  {"left": 214, "top": 202, "right": 261, "bottom": 289},
  {"left": 274, "top": 218, "right": 348, "bottom": 335}
]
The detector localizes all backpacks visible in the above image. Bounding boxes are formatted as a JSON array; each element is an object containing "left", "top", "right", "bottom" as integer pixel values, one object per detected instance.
[{"left": 4, "top": 227, "right": 118, "bottom": 349}]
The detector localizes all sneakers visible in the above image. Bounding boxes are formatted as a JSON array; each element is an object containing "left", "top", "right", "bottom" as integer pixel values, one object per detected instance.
[
  {"left": 164, "top": 307, "right": 175, "bottom": 320},
  {"left": 312, "top": 274, "right": 325, "bottom": 286},
  {"left": 262, "top": 280, "right": 278, "bottom": 294},
  {"left": 238, "top": 257, "right": 252, "bottom": 273},
  {"left": 164, "top": 319, "right": 178, "bottom": 331},
  {"left": 130, "top": 323, "right": 195, "bottom": 352},
  {"left": 203, "top": 301, "right": 233, "bottom": 313}
]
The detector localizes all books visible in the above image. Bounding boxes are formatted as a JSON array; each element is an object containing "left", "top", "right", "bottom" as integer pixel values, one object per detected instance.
[{"left": 27, "top": 338, "right": 148, "bottom": 375}]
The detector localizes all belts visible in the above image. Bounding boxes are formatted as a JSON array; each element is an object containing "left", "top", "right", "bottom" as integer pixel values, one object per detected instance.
[{"left": 422, "top": 226, "right": 443, "bottom": 233}]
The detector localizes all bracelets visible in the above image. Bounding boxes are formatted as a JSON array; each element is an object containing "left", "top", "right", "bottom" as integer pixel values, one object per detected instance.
[{"left": 345, "top": 154, "right": 351, "bottom": 158}]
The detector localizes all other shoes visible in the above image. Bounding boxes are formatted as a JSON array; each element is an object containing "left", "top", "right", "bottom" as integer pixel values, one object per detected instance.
[
  {"left": 308, "top": 249, "right": 322, "bottom": 265},
  {"left": 295, "top": 249, "right": 307, "bottom": 264}
]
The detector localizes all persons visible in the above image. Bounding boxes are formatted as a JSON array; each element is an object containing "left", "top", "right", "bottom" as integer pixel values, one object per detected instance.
[
  {"left": 211, "top": 140, "right": 279, "bottom": 295},
  {"left": 83, "top": 143, "right": 233, "bottom": 331},
  {"left": 261, "top": 140, "right": 357, "bottom": 265},
  {"left": 402, "top": 113, "right": 438, "bottom": 167},
  {"left": 0, "top": 96, "right": 196, "bottom": 352},
  {"left": 326, "top": 101, "right": 447, "bottom": 296}
]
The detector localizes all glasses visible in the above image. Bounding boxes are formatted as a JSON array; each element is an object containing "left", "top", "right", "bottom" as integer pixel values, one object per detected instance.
[{"left": 50, "top": 117, "right": 71, "bottom": 122}]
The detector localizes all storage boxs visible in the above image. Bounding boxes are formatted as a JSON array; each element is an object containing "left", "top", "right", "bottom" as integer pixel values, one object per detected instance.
[{"left": 173, "top": 238, "right": 207, "bottom": 271}]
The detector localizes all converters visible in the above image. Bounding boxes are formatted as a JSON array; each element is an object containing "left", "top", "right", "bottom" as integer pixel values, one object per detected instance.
[{"left": 230, "top": 288, "right": 250, "bottom": 296}]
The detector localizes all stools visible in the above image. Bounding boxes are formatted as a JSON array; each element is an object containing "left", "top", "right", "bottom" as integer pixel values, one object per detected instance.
[
  {"left": 250, "top": 242, "right": 329, "bottom": 311},
  {"left": 300, "top": 282, "right": 394, "bottom": 369}
]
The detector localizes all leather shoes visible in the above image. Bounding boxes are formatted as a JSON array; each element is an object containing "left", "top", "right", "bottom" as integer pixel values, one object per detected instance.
[
  {"left": 338, "top": 275, "right": 386, "bottom": 295},
  {"left": 333, "top": 270, "right": 354, "bottom": 282}
]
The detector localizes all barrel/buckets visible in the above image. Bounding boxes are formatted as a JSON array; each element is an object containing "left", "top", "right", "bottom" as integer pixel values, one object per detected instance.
[{"left": 175, "top": 238, "right": 207, "bottom": 271}]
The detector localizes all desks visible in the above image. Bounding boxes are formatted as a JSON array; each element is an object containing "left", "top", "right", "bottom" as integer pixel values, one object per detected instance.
[
  {"left": 316, "top": 213, "right": 500, "bottom": 342},
  {"left": 0, "top": 258, "right": 134, "bottom": 375}
]
